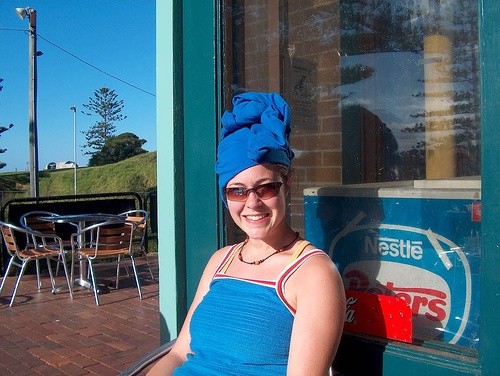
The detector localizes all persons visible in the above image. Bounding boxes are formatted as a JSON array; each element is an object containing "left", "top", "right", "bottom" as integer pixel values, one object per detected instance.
[{"left": 146, "top": 91, "right": 346, "bottom": 376}]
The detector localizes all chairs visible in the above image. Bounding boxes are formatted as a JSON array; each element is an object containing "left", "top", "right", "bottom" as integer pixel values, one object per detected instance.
[
  {"left": 116, "top": 329, "right": 333, "bottom": 376},
  {"left": 70, "top": 220, "right": 143, "bottom": 306},
  {"left": 20, "top": 211, "right": 80, "bottom": 295},
  {"left": 87, "top": 210, "right": 155, "bottom": 282},
  {"left": 0, "top": 221, "right": 74, "bottom": 307}
]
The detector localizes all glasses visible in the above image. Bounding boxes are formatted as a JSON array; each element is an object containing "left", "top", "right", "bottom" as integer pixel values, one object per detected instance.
[{"left": 223, "top": 180, "right": 285, "bottom": 202}]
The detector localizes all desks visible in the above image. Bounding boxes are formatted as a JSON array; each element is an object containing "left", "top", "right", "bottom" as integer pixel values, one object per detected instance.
[{"left": 37, "top": 214, "right": 127, "bottom": 297}]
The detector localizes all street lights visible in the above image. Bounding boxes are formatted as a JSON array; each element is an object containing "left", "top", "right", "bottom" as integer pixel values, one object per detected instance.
[{"left": 69, "top": 106, "right": 77, "bottom": 202}]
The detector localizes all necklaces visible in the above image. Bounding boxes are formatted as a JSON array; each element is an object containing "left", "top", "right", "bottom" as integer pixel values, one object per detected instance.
[{"left": 238, "top": 232, "right": 299, "bottom": 265}]
